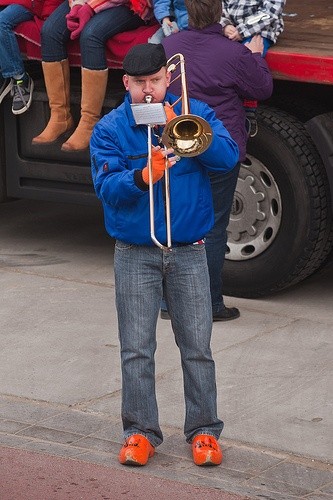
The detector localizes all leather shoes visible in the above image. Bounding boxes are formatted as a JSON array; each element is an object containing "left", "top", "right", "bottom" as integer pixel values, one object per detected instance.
[
  {"left": 161, "top": 308, "right": 170, "bottom": 320},
  {"left": 211, "top": 307, "right": 240, "bottom": 322}
]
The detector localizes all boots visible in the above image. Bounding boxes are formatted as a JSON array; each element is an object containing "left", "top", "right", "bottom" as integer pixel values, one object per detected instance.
[
  {"left": 61, "top": 66, "right": 109, "bottom": 153},
  {"left": 32, "top": 60, "right": 76, "bottom": 143}
]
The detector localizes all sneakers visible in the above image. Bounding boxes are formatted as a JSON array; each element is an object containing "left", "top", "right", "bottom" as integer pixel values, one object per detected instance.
[
  {"left": 192, "top": 433, "right": 221, "bottom": 466},
  {"left": 119, "top": 435, "right": 154, "bottom": 466},
  {"left": 0, "top": 75, "right": 15, "bottom": 105},
  {"left": 12, "top": 73, "right": 34, "bottom": 114},
  {"left": 245, "top": 112, "right": 258, "bottom": 136}
]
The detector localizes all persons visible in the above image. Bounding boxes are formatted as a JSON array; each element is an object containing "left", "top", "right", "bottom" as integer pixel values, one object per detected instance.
[
  {"left": 0, "top": 0, "right": 64, "bottom": 114},
  {"left": 32, "top": 0, "right": 151, "bottom": 152},
  {"left": 90, "top": 43, "right": 240, "bottom": 466},
  {"left": 219, "top": 0, "right": 286, "bottom": 137},
  {"left": 160, "top": 0, "right": 273, "bottom": 321},
  {"left": 149, "top": 0, "right": 188, "bottom": 44}
]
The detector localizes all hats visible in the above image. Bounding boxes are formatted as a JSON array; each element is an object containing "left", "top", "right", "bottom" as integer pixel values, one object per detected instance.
[{"left": 123, "top": 44, "right": 167, "bottom": 76}]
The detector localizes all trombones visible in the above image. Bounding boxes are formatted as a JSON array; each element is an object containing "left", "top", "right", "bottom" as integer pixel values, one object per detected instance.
[{"left": 144, "top": 53, "right": 212, "bottom": 251}]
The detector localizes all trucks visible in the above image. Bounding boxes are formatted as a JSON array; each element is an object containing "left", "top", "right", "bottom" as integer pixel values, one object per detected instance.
[{"left": 0, "top": 0, "right": 333, "bottom": 299}]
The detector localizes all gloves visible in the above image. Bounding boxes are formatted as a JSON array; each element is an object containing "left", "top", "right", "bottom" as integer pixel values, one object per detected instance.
[{"left": 66, "top": 5, "right": 93, "bottom": 39}]
